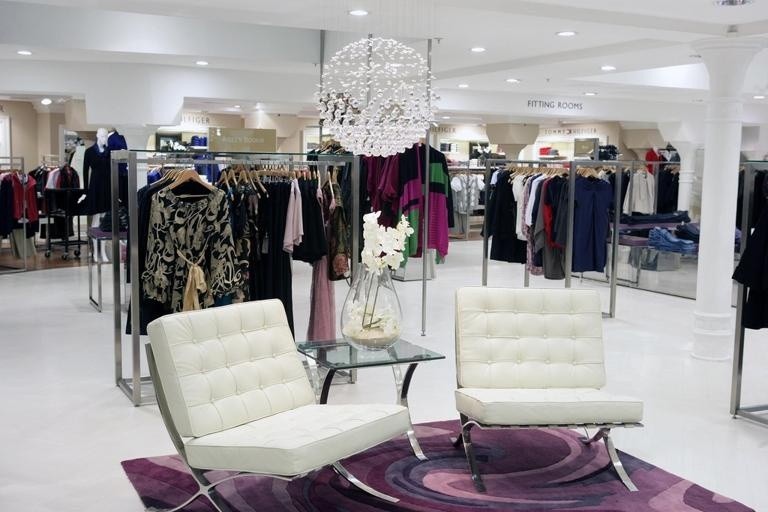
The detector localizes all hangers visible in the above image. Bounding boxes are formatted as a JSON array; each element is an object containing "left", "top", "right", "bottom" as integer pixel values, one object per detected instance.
[
  {"left": 492, "top": 163, "right": 613, "bottom": 189},
  {"left": 141, "top": 155, "right": 224, "bottom": 199},
  {"left": 216, "top": 162, "right": 343, "bottom": 206}
]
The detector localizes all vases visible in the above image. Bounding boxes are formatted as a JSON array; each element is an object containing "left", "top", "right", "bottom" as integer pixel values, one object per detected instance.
[{"left": 341, "top": 264, "right": 406, "bottom": 351}]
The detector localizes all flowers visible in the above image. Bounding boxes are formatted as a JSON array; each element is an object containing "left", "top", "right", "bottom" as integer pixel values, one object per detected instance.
[{"left": 361, "top": 211, "right": 415, "bottom": 330}]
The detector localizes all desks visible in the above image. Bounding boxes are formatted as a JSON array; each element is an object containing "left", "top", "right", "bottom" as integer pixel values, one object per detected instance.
[{"left": 296, "top": 337, "right": 445, "bottom": 461}]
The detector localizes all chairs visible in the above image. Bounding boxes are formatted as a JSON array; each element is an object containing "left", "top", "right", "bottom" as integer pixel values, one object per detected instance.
[
  {"left": 145, "top": 298, "right": 411, "bottom": 512},
  {"left": 454, "top": 285, "right": 643, "bottom": 495}
]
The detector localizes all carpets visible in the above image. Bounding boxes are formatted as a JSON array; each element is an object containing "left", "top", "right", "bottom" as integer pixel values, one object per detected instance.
[{"left": 122, "top": 420, "right": 756, "bottom": 511}]
